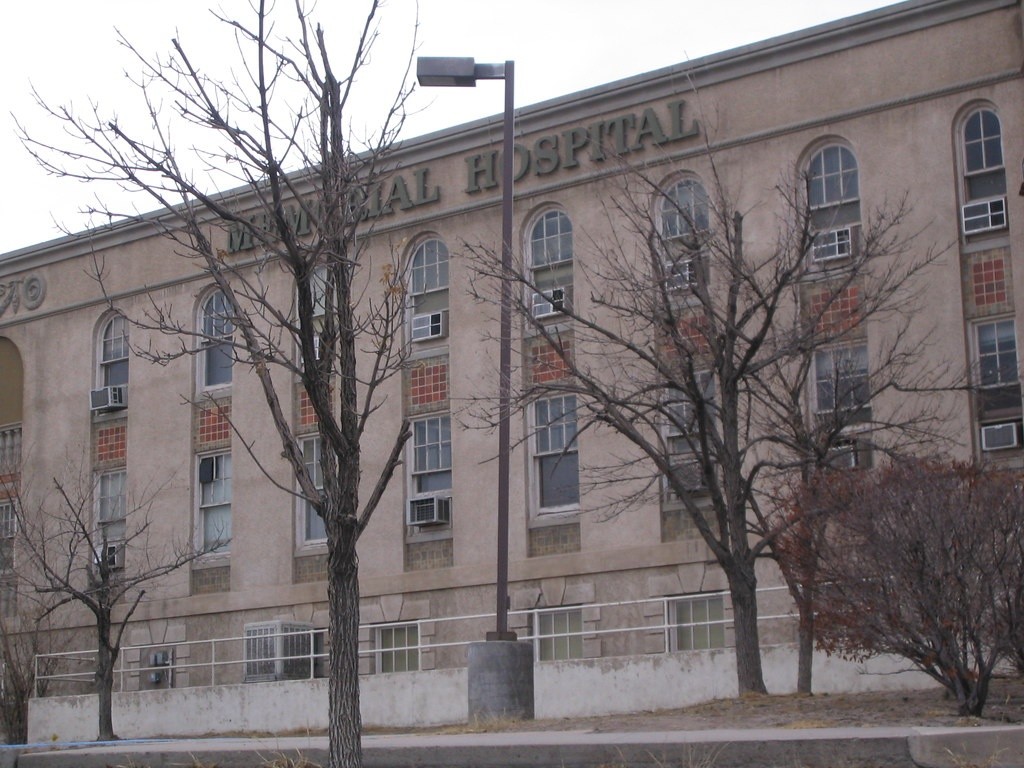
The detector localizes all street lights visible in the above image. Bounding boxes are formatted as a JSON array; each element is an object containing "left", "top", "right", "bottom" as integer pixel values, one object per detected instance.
[{"left": 414, "top": 54, "right": 536, "bottom": 724}]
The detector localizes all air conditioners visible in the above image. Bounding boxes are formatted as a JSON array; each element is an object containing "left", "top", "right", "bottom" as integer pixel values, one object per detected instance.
[
  {"left": 979, "top": 419, "right": 1021, "bottom": 451},
  {"left": 90, "top": 385, "right": 128, "bottom": 412},
  {"left": 405, "top": 496, "right": 449, "bottom": 526},
  {"left": 106, "top": 544, "right": 126, "bottom": 568},
  {"left": 822, "top": 445, "right": 857, "bottom": 473}
]
